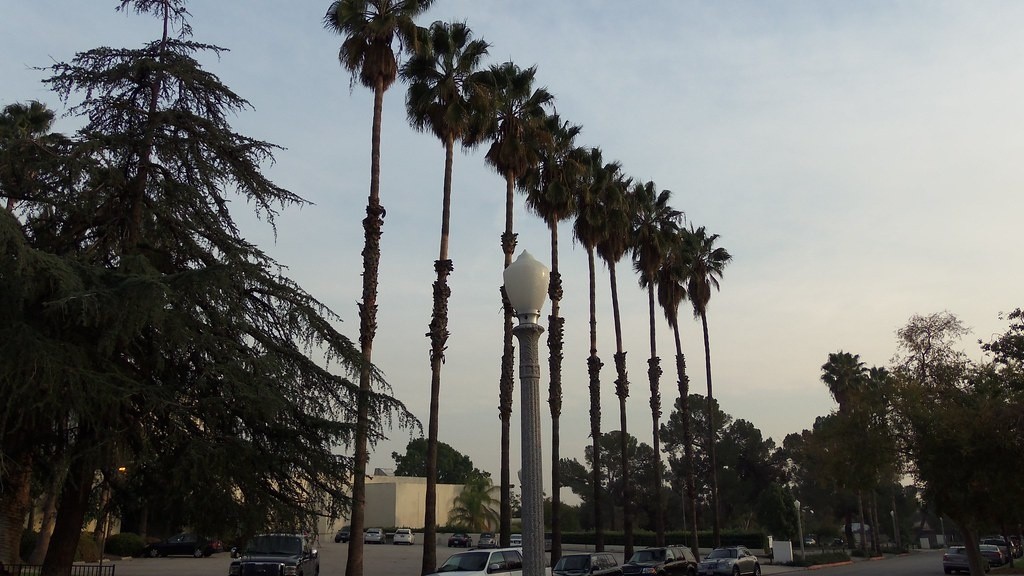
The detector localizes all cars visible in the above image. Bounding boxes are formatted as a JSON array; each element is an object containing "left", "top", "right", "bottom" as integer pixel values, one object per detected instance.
[
  {"left": 478, "top": 533, "right": 496, "bottom": 549},
  {"left": 943, "top": 546, "right": 970, "bottom": 576},
  {"left": 393, "top": 529, "right": 416, "bottom": 546},
  {"left": 365, "top": 528, "right": 387, "bottom": 544},
  {"left": 448, "top": 533, "right": 473, "bottom": 548},
  {"left": 805, "top": 538, "right": 816, "bottom": 546},
  {"left": 980, "top": 535, "right": 1024, "bottom": 563},
  {"left": 979, "top": 545, "right": 1004, "bottom": 566},
  {"left": 510, "top": 533, "right": 522, "bottom": 547},
  {"left": 335, "top": 525, "right": 351, "bottom": 543},
  {"left": 697, "top": 545, "right": 762, "bottom": 576},
  {"left": 229, "top": 532, "right": 319, "bottom": 576},
  {"left": 142, "top": 531, "right": 224, "bottom": 558},
  {"left": 552, "top": 552, "right": 624, "bottom": 576}
]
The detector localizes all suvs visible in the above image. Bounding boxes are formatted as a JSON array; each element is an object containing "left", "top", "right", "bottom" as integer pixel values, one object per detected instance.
[
  {"left": 621, "top": 544, "right": 698, "bottom": 576},
  {"left": 427, "top": 546, "right": 524, "bottom": 576}
]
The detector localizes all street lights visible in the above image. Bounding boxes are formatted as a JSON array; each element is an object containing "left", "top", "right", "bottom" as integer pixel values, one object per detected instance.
[
  {"left": 793, "top": 499, "right": 805, "bottom": 562},
  {"left": 501, "top": 248, "right": 551, "bottom": 576},
  {"left": 890, "top": 510, "right": 897, "bottom": 553},
  {"left": 940, "top": 517, "right": 946, "bottom": 546}
]
